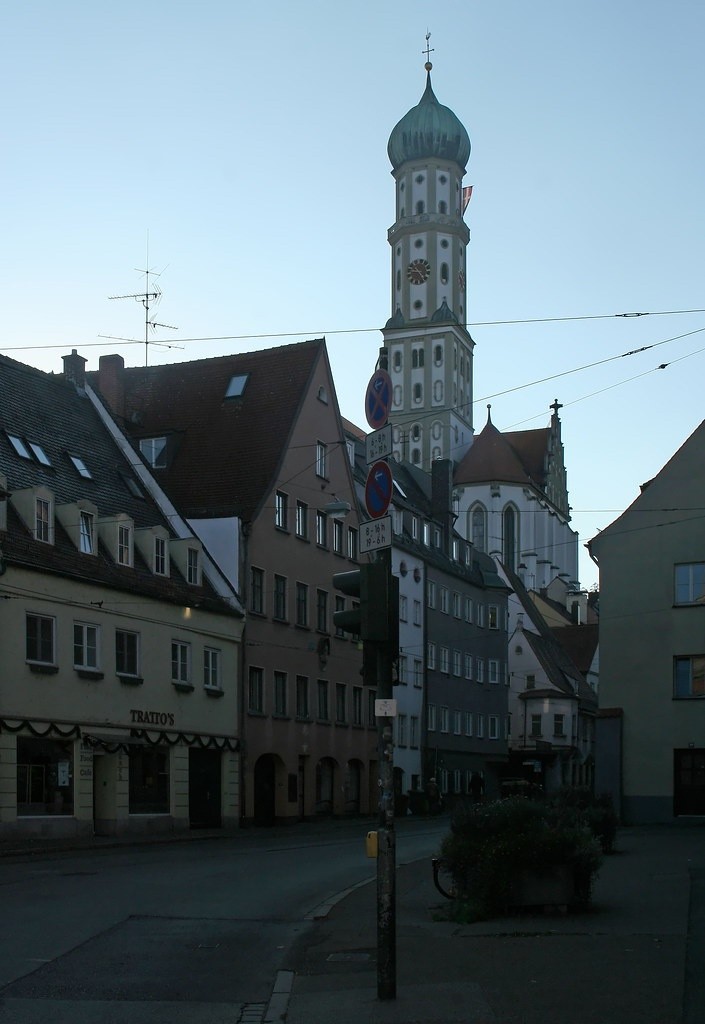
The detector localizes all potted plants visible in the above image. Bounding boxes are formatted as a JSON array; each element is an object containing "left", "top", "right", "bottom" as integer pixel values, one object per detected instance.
[{"left": 428, "top": 782, "right": 621, "bottom": 914}]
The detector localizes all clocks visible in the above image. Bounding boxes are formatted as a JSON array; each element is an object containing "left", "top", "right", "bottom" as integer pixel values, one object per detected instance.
[{"left": 407, "top": 259, "right": 431, "bottom": 285}]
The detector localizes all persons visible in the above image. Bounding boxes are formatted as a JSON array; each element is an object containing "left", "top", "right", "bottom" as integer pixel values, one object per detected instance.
[
  {"left": 468, "top": 771, "right": 485, "bottom": 805},
  {"left": 425, "top": 777, "right": 443, "bottom": 817}
]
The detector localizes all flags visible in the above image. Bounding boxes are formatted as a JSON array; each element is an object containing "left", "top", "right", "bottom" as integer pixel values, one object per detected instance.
[{"left": 462, "top": 186, "right": 473, "bottom": 214}]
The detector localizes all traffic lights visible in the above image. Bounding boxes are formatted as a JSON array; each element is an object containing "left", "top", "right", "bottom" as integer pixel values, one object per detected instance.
[{"left": 332, "top": 562, "right": 370, "bottom": 642}]
